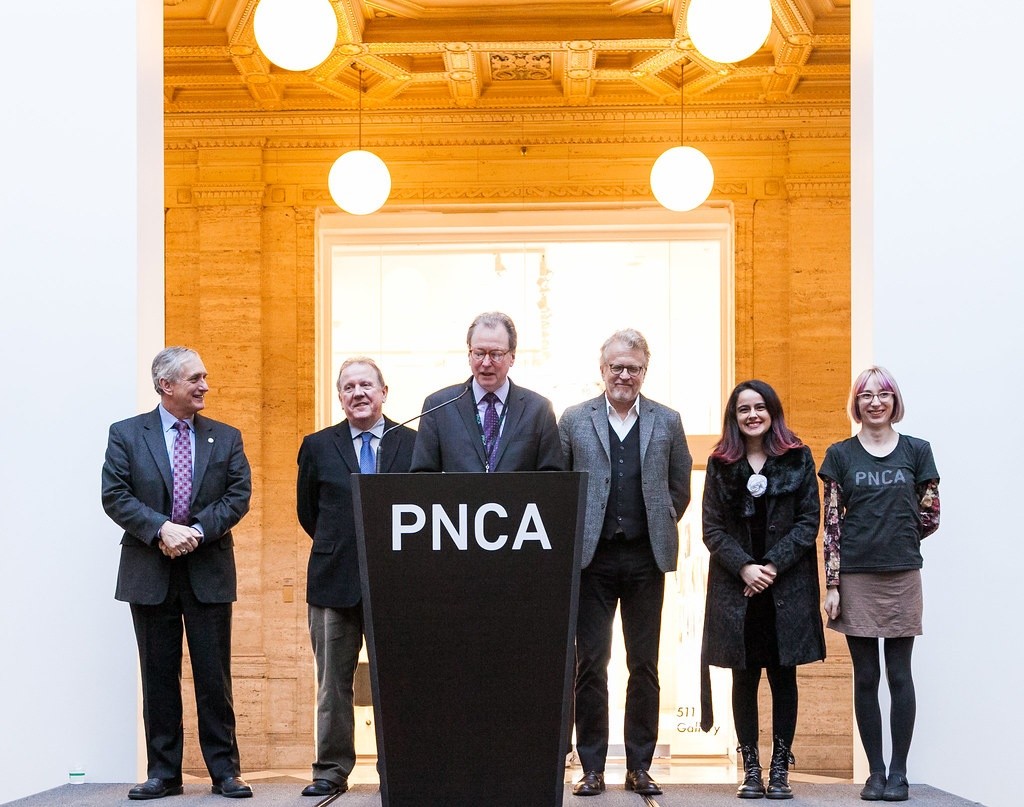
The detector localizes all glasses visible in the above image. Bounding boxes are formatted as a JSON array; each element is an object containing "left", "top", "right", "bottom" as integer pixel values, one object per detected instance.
[
  {"left": 609, "top": 363, "right": 643, "bottom": 376},
  {"left": 468, "top": 345, "right": 512, "bottom": 364},
  {"left": 857, "top": 391, "right": 895, "bottom": 402}
]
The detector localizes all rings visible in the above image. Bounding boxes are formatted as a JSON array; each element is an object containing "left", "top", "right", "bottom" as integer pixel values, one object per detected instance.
[{"left": 182, "top": 549, "right": 186, "bottom": 553}]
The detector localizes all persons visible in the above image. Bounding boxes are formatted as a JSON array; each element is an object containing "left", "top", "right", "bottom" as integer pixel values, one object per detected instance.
[
  {"left": 817, "top": 366, "right": 940, "bottom": 802},
  {"left": 102, "top": 347, "right": 253, "bottom": 800},
  {"left": 409, "top": 312, "right": 565, "bottom": 472},
  {"left": 556, "top": 328, "right": 693, "bottom": 795},
  {"left": 296, "top": 357, "right": 417, "bottom": 796},
  {"left": 700, "top": 379, "right": 826, "bottom": 799}
]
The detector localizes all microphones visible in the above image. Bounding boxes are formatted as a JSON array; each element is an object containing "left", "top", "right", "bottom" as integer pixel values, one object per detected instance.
[{"left": 376, "top": 383, "right": 472, "bottom": 473}]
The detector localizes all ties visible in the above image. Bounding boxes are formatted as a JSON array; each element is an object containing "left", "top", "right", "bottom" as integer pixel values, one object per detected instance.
[
  {"left": 171, "top": 422, "right": 193, "bottom": 526},
  {"left": 482, "top": 393, "right": 500, "bottom": 472},
  {"left": 360, "top": 433, "right": 377, "bottom": 474}
]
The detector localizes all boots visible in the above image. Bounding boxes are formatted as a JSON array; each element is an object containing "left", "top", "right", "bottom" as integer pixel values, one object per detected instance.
[
  {"left": 736, "top": 742, "right": 766, "bottom": 798},
  {"left": 767, "top": 738, "right": 796, "bottom": 799}
]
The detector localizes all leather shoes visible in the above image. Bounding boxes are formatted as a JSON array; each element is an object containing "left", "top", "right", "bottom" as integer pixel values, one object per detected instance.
[
  {"left": 885, "top": 770, "right": 909, "bottom": 801},
  {"left": 625, "top": 770, "right": 663, "bottom": 795},
  {"left": 211, "top": 776, "right": 253, "bottom": 798},
  {"left": 860, "top": 769, "right": 885, "bottom": 799},
  {"left": 573, "top": 770, "right": 605, "bottom": 795},
  {"left": 128, "top": 777, "right": 183, "bottom": 800},
  {"left": 301, "top": 780, "right": 349, "bottom": 796}
]
